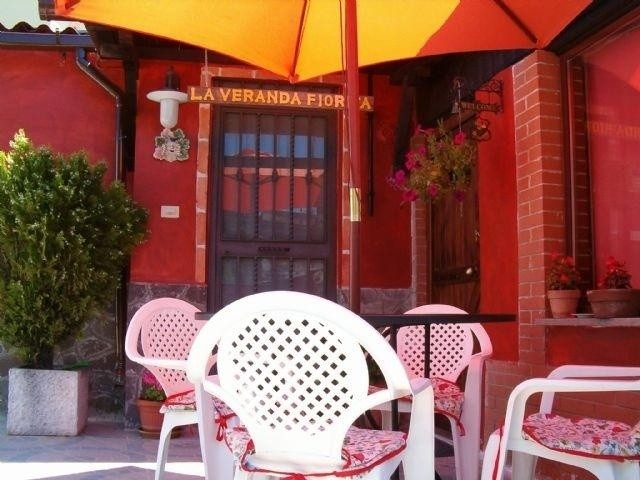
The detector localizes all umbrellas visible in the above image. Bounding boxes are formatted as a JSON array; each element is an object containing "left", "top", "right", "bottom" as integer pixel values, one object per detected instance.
[{"left": 37, "top": 0, "right": 600, "bottom": 315}]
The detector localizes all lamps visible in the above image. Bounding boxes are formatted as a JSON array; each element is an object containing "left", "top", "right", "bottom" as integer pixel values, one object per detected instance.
[{"left": 144, "top": 70, "right": 189, "bottom": 130}]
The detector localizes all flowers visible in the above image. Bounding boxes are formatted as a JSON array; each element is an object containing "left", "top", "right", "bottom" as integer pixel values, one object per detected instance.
[
  {"left": 386, "top": 115, "right": 475, "bottom": 207},
  {"left": 546, "top": 254, "right": 633, "bottom": 290}
]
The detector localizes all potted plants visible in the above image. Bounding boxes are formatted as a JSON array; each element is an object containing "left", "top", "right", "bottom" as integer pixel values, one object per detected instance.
[{"left": 0, "top": 127, "right": 152, "bottom": 439}]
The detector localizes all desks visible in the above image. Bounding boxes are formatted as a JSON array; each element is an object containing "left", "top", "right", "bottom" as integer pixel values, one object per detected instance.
[{"left": 194, "top": 311, "right": 516, "bottom": 479}]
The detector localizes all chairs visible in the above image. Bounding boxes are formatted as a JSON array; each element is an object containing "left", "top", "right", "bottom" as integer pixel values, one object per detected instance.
[
  {"left": 480, "top": 365, "right": 638, "bottom": 479},
  {"left": 123, "top": 296, "right": 218, "bottom": 479},
  {"left": 361, "top": 303, "right": 493, "bottom": 480},
  {"left": 186, "top": 291, "right": 437, "bottom": 479}
]
